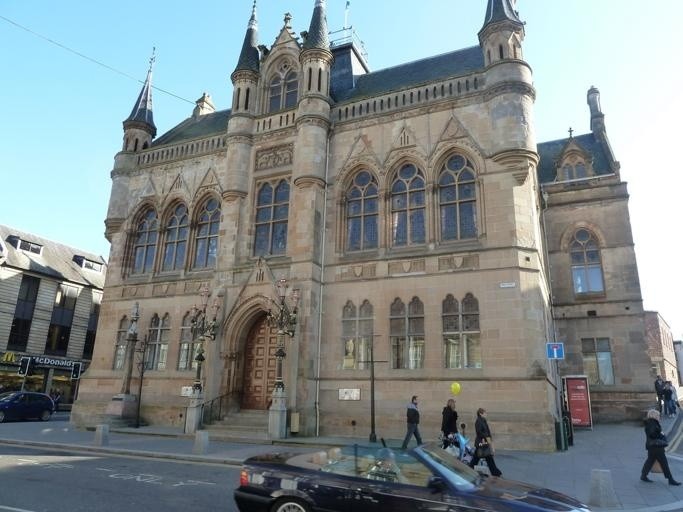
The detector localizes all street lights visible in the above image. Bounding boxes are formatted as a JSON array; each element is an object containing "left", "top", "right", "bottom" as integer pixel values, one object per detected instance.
[
  {"left": 265, "top": 278, "right": 302, "bottom": 440},
  {"left": 184, "top": 283, "right": 227, "bottom": 434},
  {"left": 120, "top": 301, "right": 142, "bottom": 396}
]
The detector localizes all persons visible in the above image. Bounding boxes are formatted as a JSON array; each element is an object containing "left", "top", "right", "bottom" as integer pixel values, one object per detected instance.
[
  {"left": 640, "top": 409, "right": 682, "bottom": 487},
  {"left": 402, "top": 396, "right": 423, "bottom": 449},
  {"left": 0, "top": 381, "right": 16, "bottom": 394},
  {"left": 366, "top": 446, "right": 408, "bottom": 483},
  {"left": 50, "top": 386, "right": 61, "bottom": 414},
  {"left": 441, "top": 398, "right": 459, "bottom": 450},
  {"left": 467, "top": 407, "right": 503, "bottom": 478},
  {"left": 655, "top": 375, "right": 680, "bottom": 418}
]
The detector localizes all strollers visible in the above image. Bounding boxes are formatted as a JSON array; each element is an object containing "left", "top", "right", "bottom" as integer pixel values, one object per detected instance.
[{"left": 436, "top": 430, "right": 486, "bottom": 465}]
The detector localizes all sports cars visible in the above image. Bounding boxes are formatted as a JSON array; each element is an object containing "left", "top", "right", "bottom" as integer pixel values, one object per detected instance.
[{"left": 227, "top": 436, "right": 598, "bottom": 512}]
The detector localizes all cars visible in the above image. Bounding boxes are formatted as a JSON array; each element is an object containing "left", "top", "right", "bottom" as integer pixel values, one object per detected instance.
[{"left": 0, "top": 391, "right": 56, "bottom": 423}]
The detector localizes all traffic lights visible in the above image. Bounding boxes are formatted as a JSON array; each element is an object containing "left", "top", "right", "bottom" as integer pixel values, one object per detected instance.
[
  {"left": 27, "top": 358, "right": 37, "bottom": 376},
  {"left": 71, "top": 362, "right": 82, "bottom": 379},
  {"left": 17, "top": 356, "right": 30, "bottom": 376}
]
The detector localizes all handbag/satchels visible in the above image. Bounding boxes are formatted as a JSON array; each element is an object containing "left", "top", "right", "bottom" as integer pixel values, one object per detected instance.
[
  {"left": 477, "top": 442, "right": 490, "bottom": 457},
  {"left": 649, "top": 439, "right": 668, "bottom": 448},
  {"left": 650, "top": 459, "right": 663, "bottom": 473}
]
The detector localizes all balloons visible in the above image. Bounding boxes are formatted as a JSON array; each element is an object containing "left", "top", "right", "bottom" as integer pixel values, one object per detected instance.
[{"left": 451, "top": 382, "right": 461, "bottom": 395}]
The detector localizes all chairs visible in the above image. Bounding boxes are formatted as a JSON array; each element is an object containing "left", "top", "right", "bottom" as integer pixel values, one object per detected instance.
[
  {"left": 312, "top": 451, "right": 327, "bottom": 467},
  {"left": 358, "top": 454, "right": 376, "bottom": 478},
  {"left": 329, "top": 448, "right": 343, "bottom": 462}
]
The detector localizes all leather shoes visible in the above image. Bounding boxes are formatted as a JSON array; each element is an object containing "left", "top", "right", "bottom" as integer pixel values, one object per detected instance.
[
  {"left": 668, "top": 480, "right": 681, "bottom": 485},
  {"left": 640, "top": 476, "right": 652, "bottom": 482}
]
